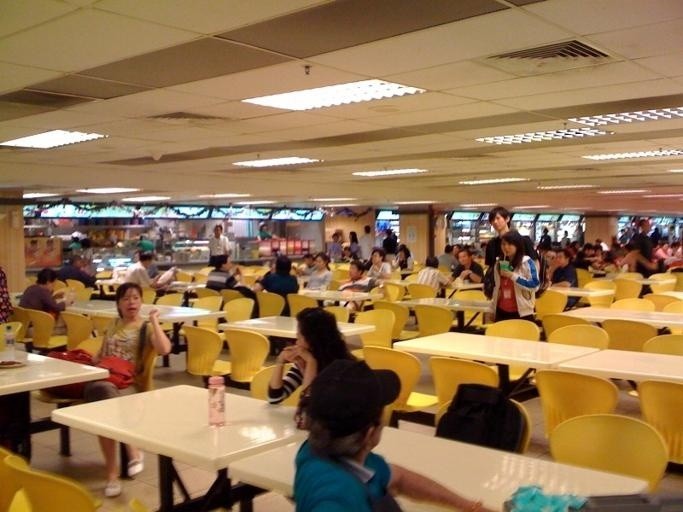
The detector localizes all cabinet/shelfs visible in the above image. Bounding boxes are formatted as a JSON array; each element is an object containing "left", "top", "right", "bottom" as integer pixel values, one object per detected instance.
[
  {"left": 256, "top": 238, "right": 315, "bottom": 257},
  {"left": 24, "top": 218, "right": 151, "bottom": 253},
  {"left": 171, "top": 240, "right": 240, "bottom": 264}
]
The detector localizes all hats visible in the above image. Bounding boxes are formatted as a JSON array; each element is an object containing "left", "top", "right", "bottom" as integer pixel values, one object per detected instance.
[{"left": 300, "top": 357, "right": 402, "bottom": 439}]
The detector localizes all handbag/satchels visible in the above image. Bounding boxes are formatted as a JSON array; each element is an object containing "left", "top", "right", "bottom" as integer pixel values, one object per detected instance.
[{"left": 96, "top": 321, "right": 150, "bottom": 386}]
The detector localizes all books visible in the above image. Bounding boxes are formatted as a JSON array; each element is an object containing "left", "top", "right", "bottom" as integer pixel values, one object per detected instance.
[{"left": 156, "top": 266, "right": 176, "bottom": 288}]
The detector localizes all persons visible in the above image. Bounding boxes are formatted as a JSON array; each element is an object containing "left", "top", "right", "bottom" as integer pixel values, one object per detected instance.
[
  {"left": 263, "top": 258, "right": 277, "bottom": 278},
  {"left": 478, "top": 243, "right": 488, "bottom": 258},
  {"left": 83, "top": 282, "right": 172, "bottom": 499},
  {"left": 123, "top": 251, "right": 169, "bottom": 289},
  {"left": 43, "top": 239, "right": 56, "bottom": 266},
  {"left": 256, "top": 256, "right": 299, "bottom": 316},
  {"left": 383, "top": 229, "right": 398, "bottom": 261},
  {"left": 205, "top": 254, "right": 241, "bottom": 293},
  {"left": 349, "top": 232, "right": 361, "bottom": 259},
  {"left": 451, "top": 243, "right": 462, "bottom": 258},
  {"left": 24, "top": 240, "right": 41, "bottom": 267},
  {"left": 541, "top": 217, "right": 682, "bottom": 311},
  {"left": 328, "top": 232, "right": 344, "bottom": 263},
  {"left": 392, "top": 243, "right": 414, "bottom": 295},
  {"left": 368, "top": 249, "right": 392, "bottom": 280},
  {"left": 437, "top": 244, "right": 459, "bottom": 269},
  {"left": 0, "top": 268, "right": 16, "bottom": 324},
  {"left": 484, "top": 207, "right": 541, "bottom": 303},
  {"left": 293, "top": 358, "right": 403, "bottom": 512},
  {"left": 493, "top": 230, "right": 540, "bottom": 323},
  {"left": 208, "top": 225, "right": 230, "bottom": 271},
  {"left": 137, "top": 235, "right": 157, "bottom": 256},
  {"left": 292, "top": 253, "right": 316, "bottom": 287},
  {"left": 449, "top": 249, "right": 484, "bottom": 283},
  {"left": 319, "top": 261, "right": 376, "bottom": 323},
  {"left": 68, "top": 228, "right": 82, "bottom": 257},
  {"left": 56, "top": 255, "right": 99, "bottom": 290},
  {"left": 75, "top": 238, "right": 93, "bottom": 264},
  {"left": 18, "top": 268, "right": 76, "bottom": 338},
  {"left": 359, "top": 225, "right": 376, "bottom": 263},
  {"left": 417, "top": 256, "right": 452, "bottom": 298},
  {"left": 259, "top": 224, "right": 272, "bottom": 241},
  {"left": 306, "top": 253, "right": 332, "bottom": 290},
  {"left": 267, "top": 307, "right": 358, "bottom": 429}
]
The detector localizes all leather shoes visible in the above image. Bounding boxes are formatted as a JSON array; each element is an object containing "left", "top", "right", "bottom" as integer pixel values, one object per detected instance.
[
  {"left": 104, "top": 477, "right": 123, "bottom": 499},
  {"left": 126, "top": 451, "right": 145, "bottom": 478}
]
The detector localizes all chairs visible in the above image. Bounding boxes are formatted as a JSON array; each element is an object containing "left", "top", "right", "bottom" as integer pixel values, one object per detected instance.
[
  {"left": 179, "top": 296, "right": 223, "bottom": 336},
  {"left": 0, "top": 321, "right": 24, "bottom": 349},
  {"left": 413, "top": 304, "right": 453, "bottom": 337},
  {"left": 576, "top": 266, "right": 683, "bottom": 313},
  {"left": 9, "top": 307, "right": 34, "bottom": 353},
  {"left": 324, "top": 307, "right": 350, "bottom": 324},
  {"left": 0, "top": 456, "right": 101, "bottom": 512},
  {"left": 288, "top": 294, "right": 317, "bottom": 318},
  {"left": 451, "top": 291, "right": 486, "bottom": 330},
  {"left": 428, "top": 357, "right": 499, "bottom": 406},
  {"left": 256, "top": 292, "right": 285, "bottom": 318},
  {"left": 363, "top": 345, "right": 440, "bottom": 428},
  {"left": 32, "top": 337, "right": 102, "bottom": 403},
  {"left": 91, "top": 316, "right": 117, "bottom": 335},
  {"left": 223, "top": 328, "right": 270, "bottom": 390},
  {"left": 27, "top": 265, "right": 270, "bottom": 299},
  {"left": 349, "top": 309, "right": 394, "bottom": 361},
  {"left": 535, "top": 289, "right": 568, "bottom": 321},
  {"left": 534, "top": 368, "right": 619, "bottom": 442},
  {"left": 632, "top": 334, "right": 683, "bottom": 398},
  {"left": 637, "top": 380, "right": 683, "bottom": 465},
  {"left": 291, "top": 259, "right": 489, "bottom": 301},
  {"left": 529, "top": 324, "right": 609, "bottom": 385},
  {"left": 435, "top": 395, "right": 530, "bottom": 455},
  {"left": 217, "top": 298, "right": 254, "bottom": 340},
  {"left": 485, "top": 320, "right": 540, "bottom": 382},
  {"left": 542, "top": 314, "right": 591, "bottom": 343},
  {"left": 21, "top": 309, "right": 69, "bottom": 357},
  {"left": 181, "top": 324, "right": 232, "bottom": 388},
  {"left": 59, "top": 312, "right": 94, "bottom": 352},
  {"left": 155, "top": 293, "right": 183, "bottom": 332},
  {"left": 550, "top": 414, "right": 669, "bottom": 493},
  {"left": 373, "top": 301, "right": 420, "bottom": 341},
  {"left": 251, "top": 364, "right": 303, "bottom": 408}
]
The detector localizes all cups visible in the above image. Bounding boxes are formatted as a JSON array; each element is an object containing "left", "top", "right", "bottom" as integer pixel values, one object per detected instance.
[
  {"left": 500, "top": 261, "right": 509, "bottom": 271},
  {"left": 206, "top": 376, "right": 228, "bottom": 427}
]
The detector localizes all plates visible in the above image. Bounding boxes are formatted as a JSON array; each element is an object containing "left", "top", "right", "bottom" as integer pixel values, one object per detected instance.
[{"left": 0, "top": 360, "right": 27, "bottom": 369}]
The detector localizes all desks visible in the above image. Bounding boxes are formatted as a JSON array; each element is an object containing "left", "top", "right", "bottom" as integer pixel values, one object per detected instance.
[
  {"left": 218, "top": 316, "right": 376, "bottom": 363},
  {"left": 98, "top": 304, "right": 228, "bottom": 368},
  {"left": 395, "top": 298, "right": 492, "bottom": 334},
  {"left": 50, "top": 383, "right": 311, "bottom": 512},
  {"left": 392, "top": 331, "right": 599, "bottom": 403},
  {"left": 0, "top": 349, "right": 108, "bottom": 465},
  {"left": 553, "top": 348, "right": 683, "bottom": 388},
  {"left": 227, "top": 430, "right": 648, "bottom": 512},
  {"left": 52, "top": 300, "right": 116, "bottom": 338}
]
